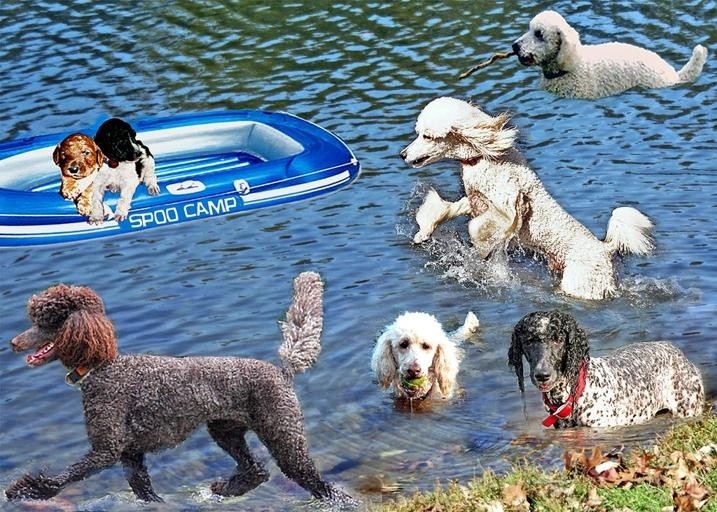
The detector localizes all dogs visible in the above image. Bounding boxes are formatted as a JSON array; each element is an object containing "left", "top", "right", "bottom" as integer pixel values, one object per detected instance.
[
  {"left": 508, "top": 310, "right": 705, "bottom": 428},
  {"left": 89, "top": 118, "right": 161, "bottom": 226},
  {"left": 511, "top": 10, "right": 709, "bottom": 100},
  {"left": 369, "top": 310, "right": 480, "bottom": 401},
  {"left": 52, "top": 132, "right": 106, "bottom": 217},
  {"left": 4, "top": 270, "right": 354, "bottom": 503},
  {"left": 397, "top": 95, "right": 658, "bottom": 301}
]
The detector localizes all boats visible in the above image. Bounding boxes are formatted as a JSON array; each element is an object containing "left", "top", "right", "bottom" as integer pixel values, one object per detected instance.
[{"left": 0, "top": 109, "right": 361, "bottom": 247}]
